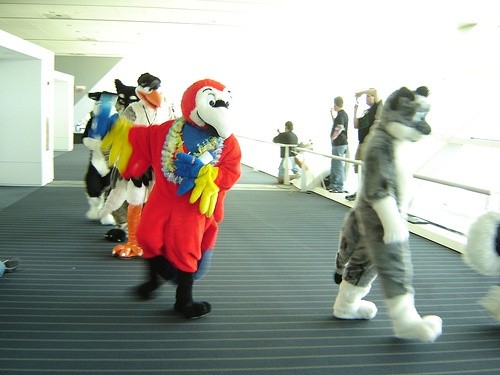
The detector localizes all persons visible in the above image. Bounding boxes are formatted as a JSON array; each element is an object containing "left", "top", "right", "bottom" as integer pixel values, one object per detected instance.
[
  {"left": 345, "top": 85, "right": 384, "bottom": 202},
  {"left": 326, "top": 96, "right": 349, "bottom": 192},
  {"left": 272, "top": 121, "right": 305, "bottom": 183}
]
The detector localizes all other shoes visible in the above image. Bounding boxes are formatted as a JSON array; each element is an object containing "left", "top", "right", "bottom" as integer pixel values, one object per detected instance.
[
  {"left": 329, "top": 189, "right": 343, "bottom": 194},
  {"left": 326, "top": 186, "right": 332, "bottom": 190},
  {"left": 345, "top": 192, "right": 357, "bottom": 201}
]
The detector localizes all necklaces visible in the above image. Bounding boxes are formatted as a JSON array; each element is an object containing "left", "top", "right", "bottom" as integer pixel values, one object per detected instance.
[{"left": 142, "top": 103, "right": 158, "bottom": 127}]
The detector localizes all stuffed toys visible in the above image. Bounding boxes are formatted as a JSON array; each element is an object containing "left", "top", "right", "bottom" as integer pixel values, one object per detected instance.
[
  {"left": 90, "top": 78, "right": 243, "bottom": 321},
  {"left": 93, "top": 79, "right": 142, "bottom": 244},
  {"left": 332, "top": 85, "right": 445, "bottom": 345},
  {"left": 81, "top": 71, "right": 177, "bottom": 258},
  {"left": 460, "top": 212, "right": 499, "bottom": 323},
  {"left": 82, "top": 90, "right": 117, "bottom": 225}
]
